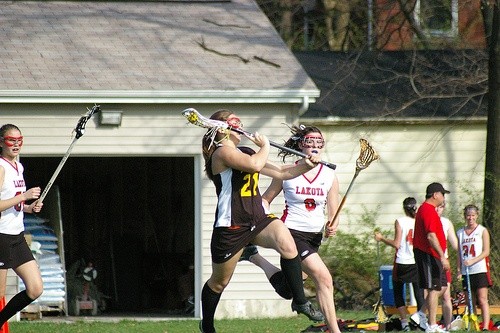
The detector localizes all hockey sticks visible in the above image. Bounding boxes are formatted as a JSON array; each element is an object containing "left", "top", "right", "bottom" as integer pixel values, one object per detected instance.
[
  {"left": 375, "top": 241, "right": 389, "bottom": 324},
  {"left": 33, "top": 103, "right": 101, "bottom": 217},
  {"left": 324, "top": 138, "right": 378, "bottom": 239},
  {"left": 463, "top": 253, "right": 480, "bottom": 331},
  {"left": 183, "top": 110, "right": 337, "bottom": 170}
]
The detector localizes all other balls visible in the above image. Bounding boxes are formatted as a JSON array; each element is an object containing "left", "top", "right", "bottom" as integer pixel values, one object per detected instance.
[{"left": 188, "top": 114, "right": 198, "bottom": 123}]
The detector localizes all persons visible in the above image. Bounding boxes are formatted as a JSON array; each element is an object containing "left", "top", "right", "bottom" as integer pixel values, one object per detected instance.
[
  {"left": 375, "top": 182, "right": 493, "bottom": 333},
  {"left": 0, "top": 124, "right": 46, "bottom": 330},
  {"left": 201, "top": 110, "right": 341, "bottom": 333}
]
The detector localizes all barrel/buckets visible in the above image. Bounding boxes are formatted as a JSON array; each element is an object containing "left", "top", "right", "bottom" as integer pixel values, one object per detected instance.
[{"left": 378, "top": 265, "right": 407, "bottom": 306}]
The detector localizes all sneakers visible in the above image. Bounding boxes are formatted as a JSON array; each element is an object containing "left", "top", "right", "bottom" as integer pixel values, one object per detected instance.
[
  {"left": 238, "top": 244, "right": 258, "bottom": 261},
  {"left": 410, "top": 311, "right": 428, "bottom": 329},
  {"left": 425, "top": 326, "right": 448, "bottom": 333},
  {"left": 291, "top": 300, "right": 325, "bottom": 323},
  {"left": 199, "top": 321, "right": 216, "bottom": 333}
]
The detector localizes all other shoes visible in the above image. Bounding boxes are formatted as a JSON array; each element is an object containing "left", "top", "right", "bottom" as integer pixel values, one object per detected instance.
[{"left": 402, "top": 325, "right": 410, "bottom": 332}]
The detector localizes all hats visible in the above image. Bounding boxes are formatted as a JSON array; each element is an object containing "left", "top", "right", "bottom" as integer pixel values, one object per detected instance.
[{"left": 426, "top": 182, "right": 450, "bottom": 194}]
